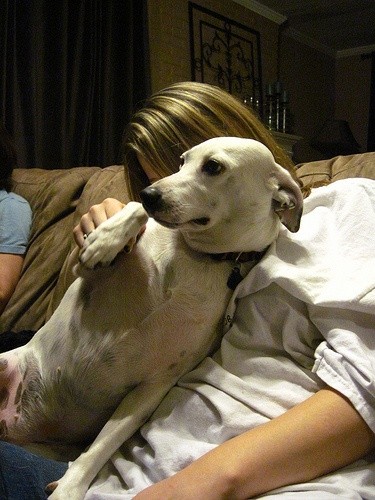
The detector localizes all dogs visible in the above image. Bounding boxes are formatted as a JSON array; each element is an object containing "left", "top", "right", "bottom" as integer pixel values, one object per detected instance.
[{"left": 1, "top": 135, "right": 305, "bottom": 500}]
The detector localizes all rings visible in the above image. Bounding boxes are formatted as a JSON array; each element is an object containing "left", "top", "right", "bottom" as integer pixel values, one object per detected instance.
[{"left": 83, "top": 229, "right": 96, "bottom": 239}]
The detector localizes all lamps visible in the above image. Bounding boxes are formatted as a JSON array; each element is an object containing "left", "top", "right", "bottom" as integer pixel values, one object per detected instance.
[{"left": 313, "top": 118, "right": 360, "bottom": 159}]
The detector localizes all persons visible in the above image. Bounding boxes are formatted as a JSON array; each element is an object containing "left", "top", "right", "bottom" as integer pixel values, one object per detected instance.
[
  {"left": 0, "top": 159, "right": 32, "bottom": 316},
  {"left": 1, "top": 80, "right": 375, "bottom": 500}
]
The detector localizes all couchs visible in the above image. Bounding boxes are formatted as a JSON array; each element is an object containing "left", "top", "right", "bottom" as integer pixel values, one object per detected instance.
[{"left": 1, "top": 150, "right": 375, "bottom": 351}]
